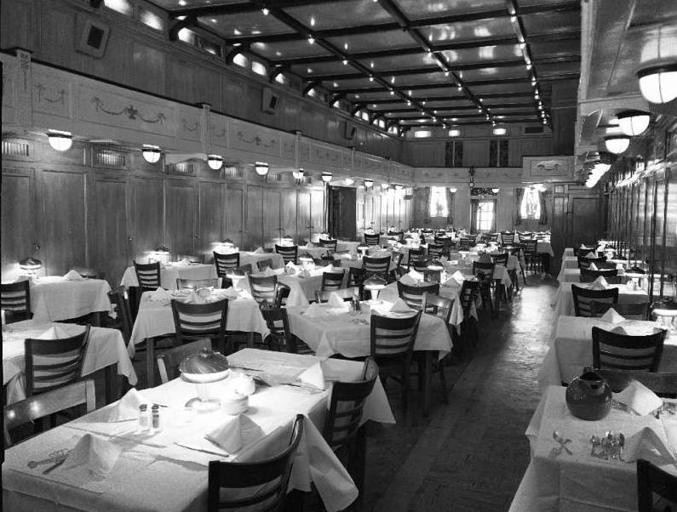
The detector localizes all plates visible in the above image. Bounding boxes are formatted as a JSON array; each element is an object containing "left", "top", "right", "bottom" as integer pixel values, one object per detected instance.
[{"left": 178, "top": 367, "right": 230, "bottom": 385}]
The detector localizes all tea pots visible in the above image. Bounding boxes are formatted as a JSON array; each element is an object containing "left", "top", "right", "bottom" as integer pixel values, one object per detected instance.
[{"left": 565, "top": 366, "right": 612, "bottom": 420}]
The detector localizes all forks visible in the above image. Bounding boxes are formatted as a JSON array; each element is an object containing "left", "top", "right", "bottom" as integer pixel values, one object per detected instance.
[{"left": 550, "top": 431, "right": 626, "bottom": 462}]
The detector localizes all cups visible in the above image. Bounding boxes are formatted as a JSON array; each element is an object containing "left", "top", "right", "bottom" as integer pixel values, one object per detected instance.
[{"left": 223, "top": 394, "right": 248, "bottom": 416}]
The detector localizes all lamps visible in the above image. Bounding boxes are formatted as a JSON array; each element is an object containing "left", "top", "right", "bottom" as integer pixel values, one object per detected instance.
[
  {"left": 253, "top": 161, "right": 403, "bottom": 191},
  {"left": 207, "top": 154, "right": 224, "bottom": 170},
  {"left": 45, "top": 128, "right": 72, "bottom": 152},
  {"left": 140, "top": 143, "right": 161, "bottom": 164},
  {"left": 584, "top": 62, "right": 677, "bottom": 192}
]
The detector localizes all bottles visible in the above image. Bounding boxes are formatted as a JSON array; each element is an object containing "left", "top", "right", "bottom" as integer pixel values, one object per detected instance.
[{"left": 138, "top": 404, "right": 161, "bottom": 434}]
